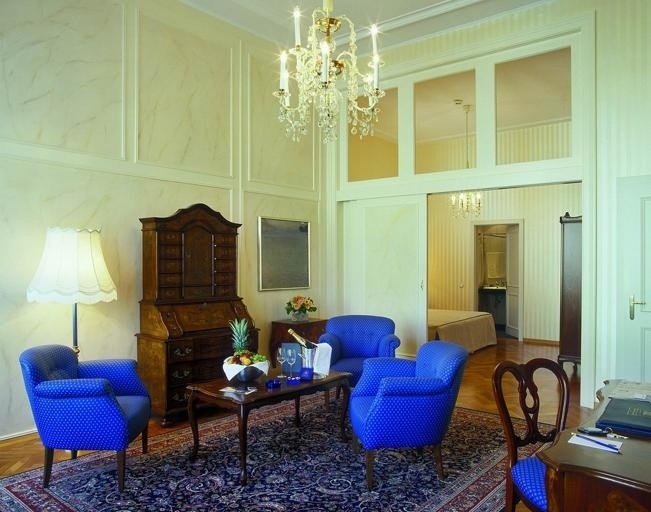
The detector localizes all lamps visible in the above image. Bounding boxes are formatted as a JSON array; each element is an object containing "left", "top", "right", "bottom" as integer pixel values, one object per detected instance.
[
  {"left": 270, "top": 1, "right": 386, "bottom": 142},
  {"left": 449, "top": 104, "right": 482, "bottom": 219},
  {"left": 26, "top": 225, "right": 117, "bottom": 362}
]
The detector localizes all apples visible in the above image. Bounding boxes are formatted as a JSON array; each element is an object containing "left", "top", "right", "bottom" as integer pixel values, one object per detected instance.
[
  {"left": 231, "top": 355, "right": 241, "bottom": 365},
  {"left": 239, "top": 353, "right": 247, "bottom": 362}
]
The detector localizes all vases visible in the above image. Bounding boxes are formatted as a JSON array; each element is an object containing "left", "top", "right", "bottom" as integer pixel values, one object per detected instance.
[{"left": 291, "top": 312, "right": 307, "bottom": 320}]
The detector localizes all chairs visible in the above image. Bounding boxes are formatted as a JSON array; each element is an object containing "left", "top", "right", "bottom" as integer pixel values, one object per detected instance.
[
  {"left": 18, "top": 344, "right": 151, "bottom": 493},
  {"left": 318, "top": 314, "right": 401, "bottom": 409},
  {"left": 348, "top": 339, "right": 469, "bottom": 489},
  {"left": 490, "top": 359, "right": 569, "bottom": 512}
]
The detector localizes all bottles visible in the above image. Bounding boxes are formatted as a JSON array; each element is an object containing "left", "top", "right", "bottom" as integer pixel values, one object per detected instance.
[{"left": 287, "top": 328, "right": 316, "bottom": 349}]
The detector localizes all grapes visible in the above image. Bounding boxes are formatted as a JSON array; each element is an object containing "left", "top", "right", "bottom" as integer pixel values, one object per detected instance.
[{"left": 249, "top": 353, "right": 266, "bottom": 363}]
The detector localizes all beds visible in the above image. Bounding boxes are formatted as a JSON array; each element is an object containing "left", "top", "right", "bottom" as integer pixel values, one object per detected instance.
[{"left": 427, "top": 308, "right": 497, "bottom": 354}]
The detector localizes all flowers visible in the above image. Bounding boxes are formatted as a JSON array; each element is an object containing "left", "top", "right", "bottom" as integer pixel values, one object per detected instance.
[{"left": 285, "top": 295, "right": 317, "bottom": 314}]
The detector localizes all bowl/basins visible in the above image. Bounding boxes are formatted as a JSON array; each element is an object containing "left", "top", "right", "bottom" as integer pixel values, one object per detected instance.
[{"left": 236, "top": 366, "right": 264, "bottom": 383}]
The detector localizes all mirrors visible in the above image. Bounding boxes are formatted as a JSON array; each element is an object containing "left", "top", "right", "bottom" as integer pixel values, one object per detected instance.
[
  {"left": 482, "top": 233, "right": 506, "bottom": 285},
  {"left": 257, "top": 215, "right": 311, "bottom": 291}
]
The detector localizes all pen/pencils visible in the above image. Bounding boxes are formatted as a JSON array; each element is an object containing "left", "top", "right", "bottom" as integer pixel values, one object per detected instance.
[{"left": 571, "top": 433, "right": 623, "bottom": 456}]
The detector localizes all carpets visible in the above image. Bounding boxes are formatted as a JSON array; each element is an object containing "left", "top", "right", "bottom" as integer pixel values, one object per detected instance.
[{"left": 0, "top": 384, "right": 568, "bottom": 511}]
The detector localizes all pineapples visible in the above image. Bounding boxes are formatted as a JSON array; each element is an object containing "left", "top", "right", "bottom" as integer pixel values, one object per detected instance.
[{"left": 226, "top": 317, "right": 261, "bottom": 356}]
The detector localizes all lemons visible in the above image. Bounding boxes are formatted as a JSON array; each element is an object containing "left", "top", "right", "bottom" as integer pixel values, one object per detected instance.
[{"left": 242, "top": 358, "right": 251, "bottom": 366}]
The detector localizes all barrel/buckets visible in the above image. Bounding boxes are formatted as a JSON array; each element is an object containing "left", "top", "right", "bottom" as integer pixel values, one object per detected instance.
[{"left": 299, "top": 345, "right": 328, "bottom": 380}]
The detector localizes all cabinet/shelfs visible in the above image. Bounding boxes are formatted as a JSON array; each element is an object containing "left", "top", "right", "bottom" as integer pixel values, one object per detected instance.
[
  {"left": 271, "top": 317, "right": 328, "bottom": 367},
  {"left": 135, "top": 204, "right": 260, "bottom": 429},
  {"left": 556, "top": 213, "right": 582, "bottom": 377}
]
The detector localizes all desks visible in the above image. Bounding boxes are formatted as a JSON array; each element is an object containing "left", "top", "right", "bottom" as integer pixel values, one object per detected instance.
[
  {"left": 536, "top": 378, "right": 651, "bottom": 512},
  {"left": 185, "top": 369, "right": 353, "bottom": 486}
]
